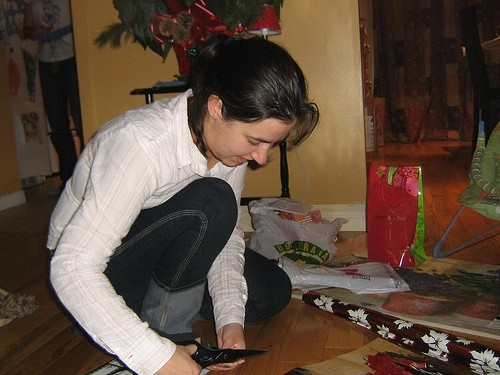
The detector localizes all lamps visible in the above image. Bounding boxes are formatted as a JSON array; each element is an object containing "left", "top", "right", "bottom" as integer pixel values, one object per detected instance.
[{"left": 247, "top": 4, "right": 280, "bottom": 40}]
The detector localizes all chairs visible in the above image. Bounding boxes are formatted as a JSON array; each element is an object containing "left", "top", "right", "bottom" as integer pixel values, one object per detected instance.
[{"left": 434, "top": 121, "right": 500, "bottom": 258}]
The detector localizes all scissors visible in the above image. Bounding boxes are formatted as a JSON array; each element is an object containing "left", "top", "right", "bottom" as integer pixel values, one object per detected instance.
[{"left": 182, "top": 339, "right": 269, "bottom": 372}]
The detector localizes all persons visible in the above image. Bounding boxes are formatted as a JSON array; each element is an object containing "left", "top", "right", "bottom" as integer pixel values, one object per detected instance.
[
  {"left": 16, "top": 2, "right": 86, "bottom": 193},
  {"left": 44, "top": 35, "right": 324, "bottom": 375}
]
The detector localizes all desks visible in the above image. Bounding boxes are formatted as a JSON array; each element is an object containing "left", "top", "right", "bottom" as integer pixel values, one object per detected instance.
[{"left": 131, "top": 81, "right": 289, "bottom": 205}]
